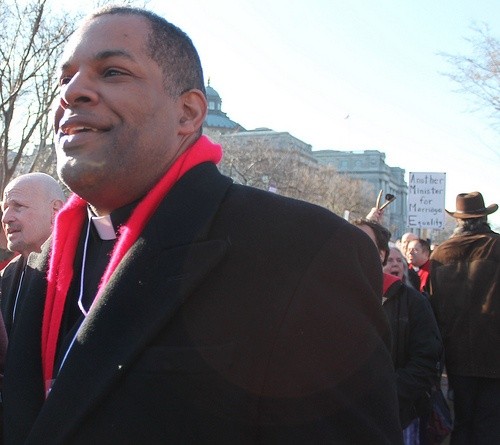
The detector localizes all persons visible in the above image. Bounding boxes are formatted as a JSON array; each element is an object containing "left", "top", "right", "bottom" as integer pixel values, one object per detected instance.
[
  {"left": 349, "top": 207, "right": 441, "bottom": 445},
  {"left": 1, "top": 8, "right": 399, "bottom": 444},
  {"left": 432, "top": 193, "right": 498, "bottom": 438},
  {"left": 382, "top": 247, "right": 441, "bottom": 357},
  {"left": 1, "top": 173, "right": 66, "bottom": 426},
  {"left": 409, "top": 240, "right": 450, "bottom": 306}
]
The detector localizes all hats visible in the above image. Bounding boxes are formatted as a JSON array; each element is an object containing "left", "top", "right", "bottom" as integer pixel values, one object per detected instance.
[{"left": 442, "top": 192, "right": 499, "bottom": 219}]
